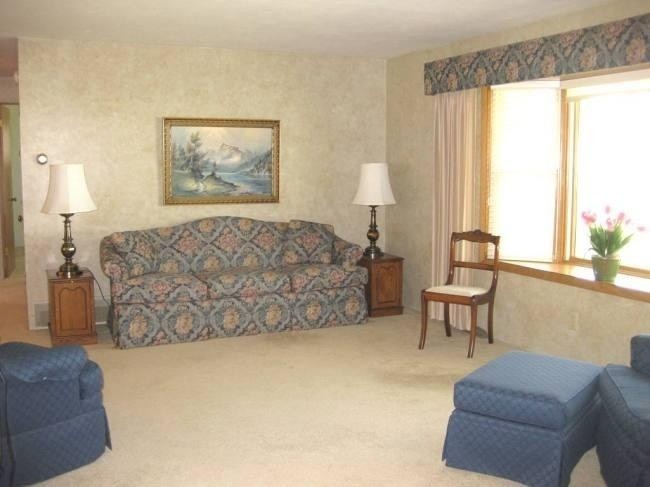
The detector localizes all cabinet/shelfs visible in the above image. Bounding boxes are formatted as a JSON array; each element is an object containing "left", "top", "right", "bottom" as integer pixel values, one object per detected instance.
[
  {"left": 45, "top": 266, "right": 98, "bottom": 346},
  {"left": 356, "top": 251, "right": 405, "bottom": 317}
]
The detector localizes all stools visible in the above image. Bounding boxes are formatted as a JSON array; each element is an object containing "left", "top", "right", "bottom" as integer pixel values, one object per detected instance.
[{"left": 442, "top": 348, "right": 605, "bottom": 485}]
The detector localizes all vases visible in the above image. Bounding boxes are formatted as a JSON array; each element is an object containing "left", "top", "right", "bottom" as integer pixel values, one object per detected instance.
[{"left": 590, "top": 256, "right": 621, "bottom": 280}]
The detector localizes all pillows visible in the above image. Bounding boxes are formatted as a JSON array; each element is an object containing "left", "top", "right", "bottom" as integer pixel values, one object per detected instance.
[
  {"left": 283, "top": 219, "right": 335, "bottom": 264},
  {"left": 111, "top": 229, "right": 162, "bottom": 278}
]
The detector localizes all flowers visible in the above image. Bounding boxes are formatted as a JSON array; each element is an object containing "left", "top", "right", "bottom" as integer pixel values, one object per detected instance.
[{"left": 580, "top": 205, "right": 646, "bottom": 258}]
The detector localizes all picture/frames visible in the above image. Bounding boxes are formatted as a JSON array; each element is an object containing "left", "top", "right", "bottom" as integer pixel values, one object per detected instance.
[{"left": 162, "top": 116, "right": 280, "bottom": 205}]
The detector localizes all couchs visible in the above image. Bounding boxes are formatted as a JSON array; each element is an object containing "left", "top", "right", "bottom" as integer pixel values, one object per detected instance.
[{"left": 99, "top": 215, "right": 369, "bottom": 350}]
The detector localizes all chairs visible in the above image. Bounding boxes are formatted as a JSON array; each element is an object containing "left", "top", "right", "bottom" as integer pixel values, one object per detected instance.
[
  {"left": 597, "top": 333, "right": 650, "bottom": 485},
  {"left": 0, "top": 340, "right": 111, "bottom": 486},
  {"left": 417, "top": 229, "right": 501, "bottom": 358}
]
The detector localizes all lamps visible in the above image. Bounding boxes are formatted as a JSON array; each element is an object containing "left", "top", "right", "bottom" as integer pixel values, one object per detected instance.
[
  {"left": 351, "top": 161, "right": 397, "bottom": 256},
  {"left": 40, "top": 162, "right": 97, "bottom": 277}
]
